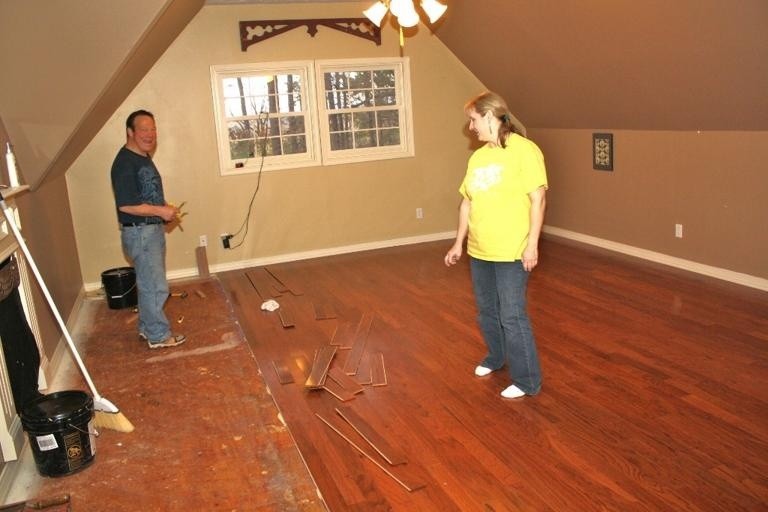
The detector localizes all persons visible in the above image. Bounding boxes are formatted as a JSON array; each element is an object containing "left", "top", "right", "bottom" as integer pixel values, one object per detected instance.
[
  {"left": 441, "top": 89, "right": 551, "bottom": 399},
  {"left": 110, "top": 109, "right": 189, "bottom": 351}
]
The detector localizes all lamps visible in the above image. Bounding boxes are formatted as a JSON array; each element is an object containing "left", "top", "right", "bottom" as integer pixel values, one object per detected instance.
[{"left": 361, "top": 0, "right": 449, "bottom": 31}]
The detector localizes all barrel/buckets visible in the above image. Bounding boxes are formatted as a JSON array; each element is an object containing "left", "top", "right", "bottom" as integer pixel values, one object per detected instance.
[
  {"left": 101, "top": 267, "right": 137, "bottom": 309},
  {"left": 21, "top": 390, "right": 98, "bottom": 477}
]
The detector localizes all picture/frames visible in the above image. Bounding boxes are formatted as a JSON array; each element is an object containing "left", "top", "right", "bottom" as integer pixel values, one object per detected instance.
[{"left": 592, "top": 133, "right": 613, "bottom": 171}]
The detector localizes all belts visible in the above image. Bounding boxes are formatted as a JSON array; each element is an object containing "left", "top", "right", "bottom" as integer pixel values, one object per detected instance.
[{"left": 121, "top": 220, "right": 165, "bottom": 229}]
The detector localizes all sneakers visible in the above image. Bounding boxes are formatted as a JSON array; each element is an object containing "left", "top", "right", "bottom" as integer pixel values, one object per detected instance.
[{"left": 139, "top": 329, "right": 186, "bottom": 349}]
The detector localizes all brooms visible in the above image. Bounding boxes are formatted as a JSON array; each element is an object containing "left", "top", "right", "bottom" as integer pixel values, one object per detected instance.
[{"left": 0, "top": 191, "right": 136, "bottom": 433}]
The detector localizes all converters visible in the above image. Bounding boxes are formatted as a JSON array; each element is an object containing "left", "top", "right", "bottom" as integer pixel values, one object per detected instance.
[{"left": 222, "top": 239, "right": 230, "bottom": 249}]
